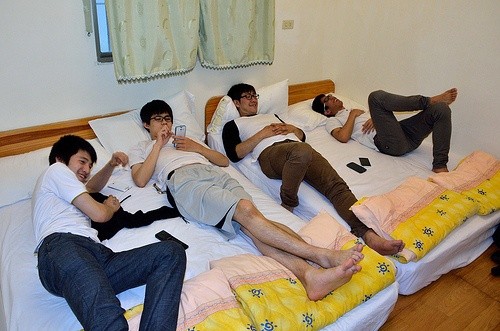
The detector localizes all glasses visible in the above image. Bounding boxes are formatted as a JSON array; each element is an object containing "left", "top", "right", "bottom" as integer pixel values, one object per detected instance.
[
  {"left": 238, "top": 94, "right": 259, "bottom": 100},
  {"left": 149, "top": 116, "right": 171, "bottom": 122},
  {"left": 323, "top": 94, "right": 332, "bottom": 111}
]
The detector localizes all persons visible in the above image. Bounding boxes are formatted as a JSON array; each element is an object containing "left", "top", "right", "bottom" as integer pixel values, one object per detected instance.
[
  {"left": 221, "top": 83, "right": 406, "bottom": 256},
  {"left": 128, "top": 99, "right": 364, "bottom": 302},
  {"left": 31, "top": 134, "right": 187, "bottom": 331},
  {"left": 312, "top": 88, "right": 458, "bottom": 174}
]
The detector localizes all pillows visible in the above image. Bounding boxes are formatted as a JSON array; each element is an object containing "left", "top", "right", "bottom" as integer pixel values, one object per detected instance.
[
  {"left": 88, "top": 91, "right": 204, "bottom": 155},
  {"left": 289, "top": 92, "right": 365, "bottom": 132},
  {"left": 207, "top": 79, "right": 289, "bottom": 134},
  {"left": 0, "top": 138, "right": 128, "bottom": 208}
]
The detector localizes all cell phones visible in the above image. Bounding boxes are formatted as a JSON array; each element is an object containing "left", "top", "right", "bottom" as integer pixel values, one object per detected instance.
[
  {"left": 359, "top": 158, "right": 371, "bottom": 166},
  {"left": 107, "top": 181, "right": 129, "bottom": 191},
  {"left": 174, "top": 125, "right": 186, "bottom": 146},
  {"left": 346, "top": 162, "right": 367, "bottom": 174}
]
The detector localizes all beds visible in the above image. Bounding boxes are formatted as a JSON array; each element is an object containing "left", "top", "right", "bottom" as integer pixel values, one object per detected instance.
[{"left": 0, "top": 80, "right": 500, "bottom": 331}]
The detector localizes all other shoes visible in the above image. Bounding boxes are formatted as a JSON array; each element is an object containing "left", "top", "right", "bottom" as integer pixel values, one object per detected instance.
[
  {"left": 491, "top": 251, "right": 500, "bottom": 264},
  {"left": 491, "top": 265, "right": 500, "bottom": 277}
]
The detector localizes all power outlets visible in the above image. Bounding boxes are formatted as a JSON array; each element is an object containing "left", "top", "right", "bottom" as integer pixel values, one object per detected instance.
[{"left": 282, "top": 20, "right": 294, "bottom": 28}]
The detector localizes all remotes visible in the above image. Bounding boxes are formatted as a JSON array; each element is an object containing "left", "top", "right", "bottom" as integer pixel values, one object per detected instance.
[{"left": 155, "top": 230, "right": 189, "bottom": 250}]
